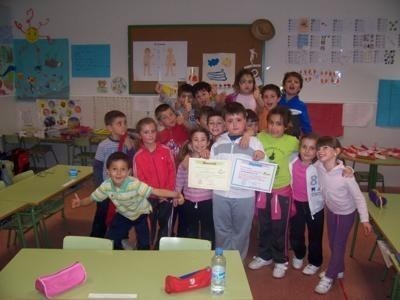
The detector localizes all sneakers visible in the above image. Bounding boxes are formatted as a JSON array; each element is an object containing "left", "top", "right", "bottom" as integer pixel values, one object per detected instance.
[
  {"left": 272, "top": 262, "right": 288, "bottom": 278},
  {"left": 318, "top": 267, "right": 344, "bottom": 280},
  {"left": 302, "top": 263, "right": 320, "bottom": 275},
  {"left": 248, "top": 255, "right": 272, "bottom": 269},
  {"left": 314, "top": 276, "right": 333, "bottom": 295},
  {"left": 292, "top": 255, "right": 304, "bottom": 269}
]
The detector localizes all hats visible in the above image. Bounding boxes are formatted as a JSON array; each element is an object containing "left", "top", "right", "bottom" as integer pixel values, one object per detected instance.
[{"left": 251, "top": 19, "right": 275, "bottom": 40}]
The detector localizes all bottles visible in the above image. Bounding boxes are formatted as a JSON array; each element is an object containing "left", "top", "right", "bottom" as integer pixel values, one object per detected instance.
[{"left": 210, "top": 247, "right": 226, "bottom": 297}]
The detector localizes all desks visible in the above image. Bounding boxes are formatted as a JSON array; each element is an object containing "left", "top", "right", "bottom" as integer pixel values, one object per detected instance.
[
  {"left": 0, "top": 164, "right": 96, "bottom": 254},
  {"left": 0, "top": 199, "right": 29, "bottom": 262},
  {"left": 350, "top": 191, "right": 399, "bottom": 300},
  {"left": 339, "top": 144, "right": 399, "bottom": 193},
  {"left": 0, "top": 248, "right": 253, "bottom": 300}
]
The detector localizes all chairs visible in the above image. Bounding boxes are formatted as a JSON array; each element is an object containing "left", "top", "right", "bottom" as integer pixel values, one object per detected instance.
[
  {"left": 159, "top": 237, "right": 212, "bottom": 251},
  {"left": 62, "top": 236, "right": 113, "bottom": 250}
]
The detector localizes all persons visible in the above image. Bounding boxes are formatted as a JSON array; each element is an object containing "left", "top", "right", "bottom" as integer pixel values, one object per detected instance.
[
  {"left": 254, "top": 84, "right": 281, "bottom": 132},
  {"left": 278, "top": 72, "right": 312, "bottom": 137},
  {"left": 174, "top": 83, "right": 193, "bottom": 126},
  {"left": 70, "top": 152, "right": 184, "bottom": 250},
  {"left": 200, "top": 102, "right": 265, "bottom": 268},
  {"left": 91, "top": 110, "right": 135, "bottom": 237},
  {"left": 244, "top": 108, "right": 259, "bottom": 134},
  {"left": 289, "top": 135, "right": 354, "bottom": 275},
  {"left": 192, "top": 81, "right": 217, "bottom": 111},
  {"left": 224, "top": 69, "right": 256, "bottom": 112},
  {"left": 312, "top": 136, "right": 371, "bottom": 295},
  {"left": 154, "top": 103, "right": 191, "bottom": 165},
  {"left": 173, "top": 127, "right": 214, "bottom": 249},
  {"left": 248, "top": 106, "right": 299, "bottom": 277},
  {"left": 132, "top": 117, "right": 176, "bottom": 246},
  {"left": 200, "top": 112, "right": 254, "bottom": 159},
  {"left": 183, "top": 106, "right": 214, "bottom": 153}
]
